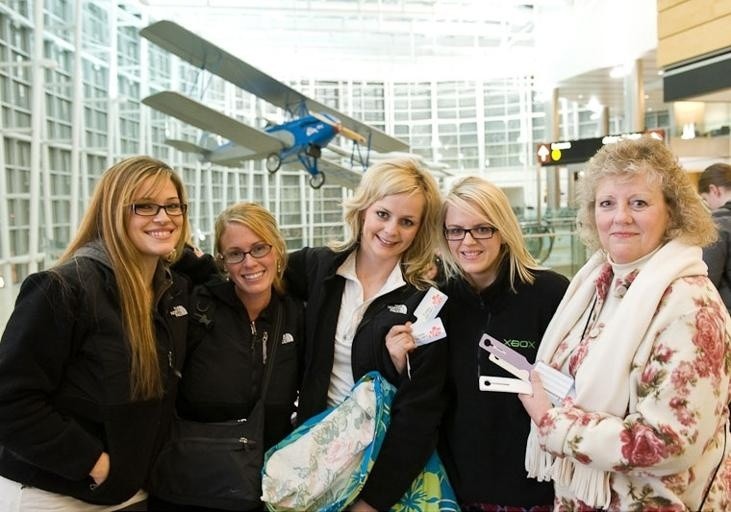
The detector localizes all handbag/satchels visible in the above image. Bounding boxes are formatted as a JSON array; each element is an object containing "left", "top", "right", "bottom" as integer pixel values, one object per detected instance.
[{"left": 153, "top": 397, "right": 276, "bottom": 511}]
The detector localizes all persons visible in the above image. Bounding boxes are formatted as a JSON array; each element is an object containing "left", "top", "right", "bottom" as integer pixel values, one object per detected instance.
[
  {"left": 518, "top": 137, "right": 731, "bottom": 511},
  {"left": 208, "top": 157, "right": 456, "bottom": 512},
  {"left": 2, "top": 154, "right": 195, "bottom": 511},
  {"left": 141, "top": 201, "right": 307, "bottom": 512},
  {"left": 695, "top": 162, "right": 731, "bottom": 316},
  {"left": 382, "top": 176, "right": 572, "bottom": 512}
]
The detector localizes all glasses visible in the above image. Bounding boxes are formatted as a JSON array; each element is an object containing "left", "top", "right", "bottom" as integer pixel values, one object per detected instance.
[
  {"left": 222, "top": 241, "right": 272, "bottom": 265},
  {"left": 124, "top": 202, "right": 189, "bottom": 216},
  {"left": 444, "top": 226, "right": 499, "bottom": 240}
]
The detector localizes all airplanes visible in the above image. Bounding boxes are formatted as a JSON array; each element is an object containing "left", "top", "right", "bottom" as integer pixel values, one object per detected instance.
[{"left": 132, "top": 17, "right": 415, "bottom": 205}]
